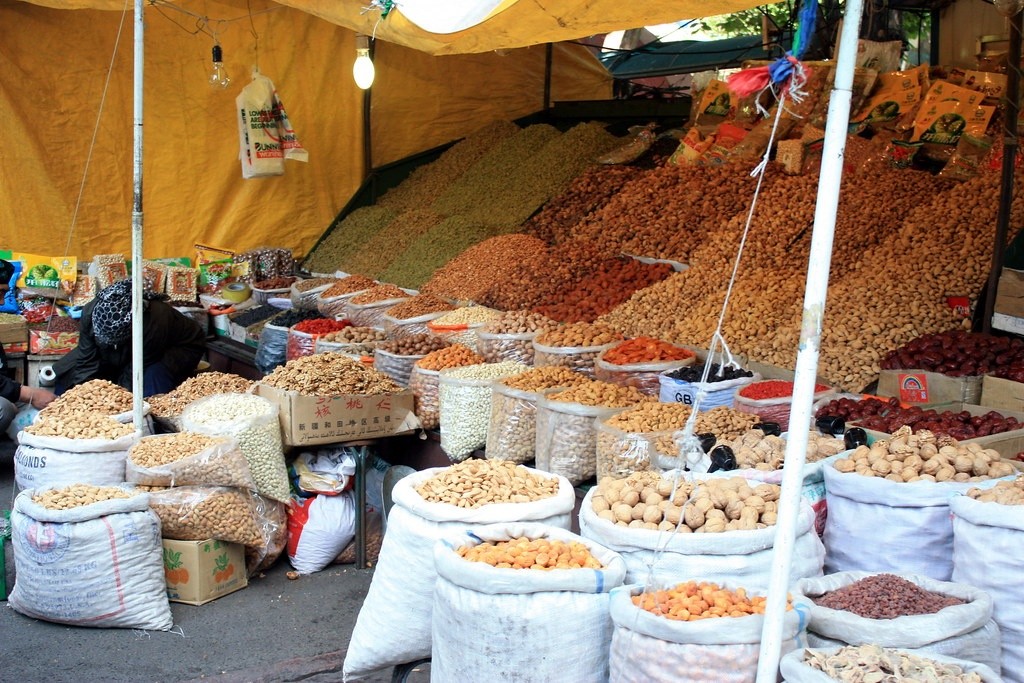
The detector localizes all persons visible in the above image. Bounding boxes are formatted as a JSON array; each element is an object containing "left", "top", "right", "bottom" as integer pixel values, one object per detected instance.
[
  {"left": 0, "top": 259, "right": 59, "bottom": 445},
  {"left": 53, "top": 279, "right": 206, "bottom": 399}
]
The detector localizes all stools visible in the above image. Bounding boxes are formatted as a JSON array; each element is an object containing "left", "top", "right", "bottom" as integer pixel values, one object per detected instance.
[{"left": 336, "top": 440, "right": 377, "bottom": 569}]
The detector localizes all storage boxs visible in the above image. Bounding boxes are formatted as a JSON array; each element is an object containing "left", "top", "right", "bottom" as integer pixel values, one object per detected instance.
[
  {"left": 981, "top": 371, "right": 1024, "bottom": 412},
  {"left": 250, "top": 382, "right": 423, "bottom": 446},
  {"left": 3, "top": 343, "right": 28, "bottom": 353},
  {"left": 0, "top": 315, "right": 28, "bottom": 344},
  {"left": 161, "top": 538, "right": 248, "bottom": 606},
  {"left": 29, "top": 329, "right": 80, "bottom": 355},
  {"left": 876, "top": 368, "right": 984, "bottom": 408},
  {"left": 230, "top": 306, "right": 283, "bottom": 343},
  {"left": 844, "top": 401, "right": 1024, "bottom": 458}
]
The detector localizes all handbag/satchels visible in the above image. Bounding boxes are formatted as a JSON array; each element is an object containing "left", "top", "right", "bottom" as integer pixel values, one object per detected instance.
[{"left": 236, "top": 76, "right": 309, "bottom": 180}]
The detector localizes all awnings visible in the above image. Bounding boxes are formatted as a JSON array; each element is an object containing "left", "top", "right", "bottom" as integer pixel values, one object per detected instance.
[{"left": 596, "top": 25, "right": 794, "bottom": 100}]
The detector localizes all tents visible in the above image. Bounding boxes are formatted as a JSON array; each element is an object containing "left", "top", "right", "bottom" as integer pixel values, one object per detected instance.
[{"left": 3, "top": 1, "right": 869, "bottom": 683}]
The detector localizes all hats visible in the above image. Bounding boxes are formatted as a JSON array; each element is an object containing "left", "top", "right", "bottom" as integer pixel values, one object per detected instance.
[{"left": 0, "top": 259, "right": 15, "bottom": 284}]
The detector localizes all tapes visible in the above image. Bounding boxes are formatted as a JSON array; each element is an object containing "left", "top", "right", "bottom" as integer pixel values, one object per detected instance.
[{"left": 220, "top": 281, "right": 251, "bottom": 303}]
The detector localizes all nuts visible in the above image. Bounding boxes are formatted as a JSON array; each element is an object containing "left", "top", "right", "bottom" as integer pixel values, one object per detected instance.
[
  {"left": 298, "top": 62, "right": 841, "bottom": 372},
  {"left": 7, "top": 268, "right": 1024, "bottom": 683},
  {"left": 815, "top": 132, "right": 1024, "bottom": 393}
]
